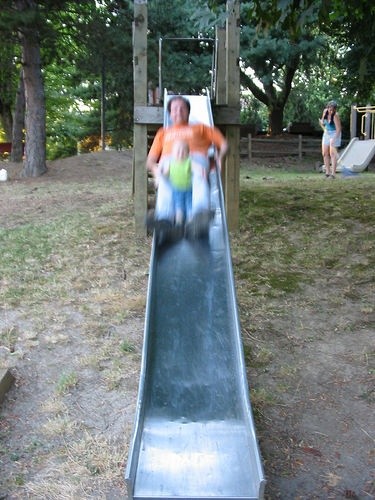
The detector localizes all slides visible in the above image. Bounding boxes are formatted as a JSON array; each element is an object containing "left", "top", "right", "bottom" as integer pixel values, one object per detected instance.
[
  {"left": 125, "top": 87, "right": 269, "bottom": 500},
  {"left": 320, "top": 138, "right": 374, "bottom": 174}
]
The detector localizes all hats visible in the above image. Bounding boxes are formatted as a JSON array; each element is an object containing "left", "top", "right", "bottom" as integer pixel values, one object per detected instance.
[{"left": 327, "top": 99, "right": 336, "bottom": 107}]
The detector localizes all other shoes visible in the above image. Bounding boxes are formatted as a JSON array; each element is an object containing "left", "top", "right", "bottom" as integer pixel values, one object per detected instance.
[
  {"left": 326, "top": 173, "right": 336, "bottom": 178},
  {"left": 184, "top": 208, "right": 214, "bottom": 241},
  {"left": 147, "top": 216, "right": 182, "bottom": 242}
]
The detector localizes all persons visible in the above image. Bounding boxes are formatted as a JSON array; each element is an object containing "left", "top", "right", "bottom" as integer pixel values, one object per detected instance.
[
  {"left": 156, "top": 140, "right": 209, "bottom": 240},
  {"left": 318, "top": 100, "right": 342, "bottom": 180},
  {"left": 144, "top": 95, "right": 230, "bottom": 241}
]
